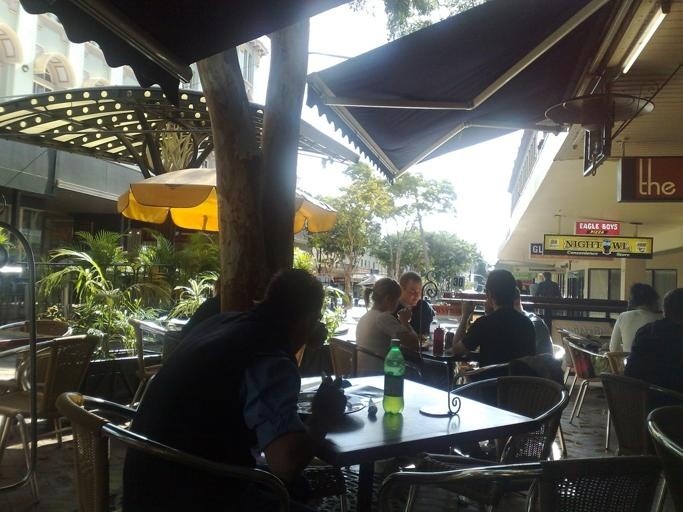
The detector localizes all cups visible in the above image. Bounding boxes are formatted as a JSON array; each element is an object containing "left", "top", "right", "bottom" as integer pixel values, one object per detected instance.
[{"left": 303, "top": 333, "right": 324, "bottom": 353}]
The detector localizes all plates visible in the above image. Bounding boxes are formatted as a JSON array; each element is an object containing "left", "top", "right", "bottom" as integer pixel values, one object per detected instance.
[{"left": 297, "top": 392, "right": 365, "bottom": 415}]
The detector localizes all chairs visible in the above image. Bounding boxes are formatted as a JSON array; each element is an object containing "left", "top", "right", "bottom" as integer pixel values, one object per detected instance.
[
  {"left": 0, "top": 335, "right": 98, "bottom": 503},
  {"left": 0, "top": 320, "right": 68, "bottom": 335},
  {"left": 303, "top": 457, "right": 347, "bottom": 512},
  {"left": 129, "top": 321, "right": 162, "bottom": 407},
  {"left": 328, "top": 337, "right": 423, "bottom": 383},
  {"left": 371, "top": 451, "right": 670, "bottom": 512},
  {"left": 54, "top": 392, "right": 293, "bottom": 512}
]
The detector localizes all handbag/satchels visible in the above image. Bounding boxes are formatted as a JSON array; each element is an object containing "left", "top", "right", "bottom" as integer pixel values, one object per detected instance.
[
  {"left": 589, "top": 356, "right": 609, "bottom": 376},
  {"left": 575, "top": 352, "right": 592, "bottom": 378}
]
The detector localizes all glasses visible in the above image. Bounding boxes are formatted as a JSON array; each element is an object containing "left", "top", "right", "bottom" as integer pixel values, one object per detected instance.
[
  {"left": 483, "top": 289, "right": 487, "bottom": 293},
  {"left": 384, "top": 278, "right": 399, "bottom": 287}
]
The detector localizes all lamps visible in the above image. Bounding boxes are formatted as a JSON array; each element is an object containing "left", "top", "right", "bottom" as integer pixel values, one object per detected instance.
[{"left": 620, "top": 0, "right": 671, "bottom": 75}]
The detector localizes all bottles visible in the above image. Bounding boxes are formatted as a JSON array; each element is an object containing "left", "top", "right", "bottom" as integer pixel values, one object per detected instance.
[
  {"left": 433, "top": 324, "right": 444, "bottom": 353},
  {"left": 382, "top": 339, "right": 405, "bottom": 413}
]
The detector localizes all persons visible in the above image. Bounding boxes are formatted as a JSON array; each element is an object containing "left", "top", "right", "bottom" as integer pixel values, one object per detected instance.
[
  {"left": 537, "top": 272, "right": 561, "bottom": 334},
  {"left": 356, "top": 277, "right": 419, "bottom": 376},
  {"left": 393, "top": 271, "right": 435, "bottom": 339},
  {"left": 609, "top": 282, "right": 663, "bottom": 365},
  {"left": 452, "top": 270, "right": 536, "bottom": 384},
  {"left": 623, "top": 287, "right": 683, "bottom": 409},
  {"left": 485, "top": 287, "right": 554, "bottom": 357},
  {"left": 531, "top": 273, "right": 545, "bottom": 314},
  {"left": 181, "top": 274, "right": 221, "bottom": 340},
  {"left": 121, "top": 268, "right": 346, "bottom": 512}
]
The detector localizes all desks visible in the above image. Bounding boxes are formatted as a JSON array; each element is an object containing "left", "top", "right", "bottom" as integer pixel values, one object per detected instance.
[{"left": 0, "top": 330, "right": 60, "bottom": 351}]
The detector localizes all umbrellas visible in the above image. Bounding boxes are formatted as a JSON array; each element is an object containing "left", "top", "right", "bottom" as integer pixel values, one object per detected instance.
[{"left": 117, "top": 167, "right": 338, "bottom": 236}]
